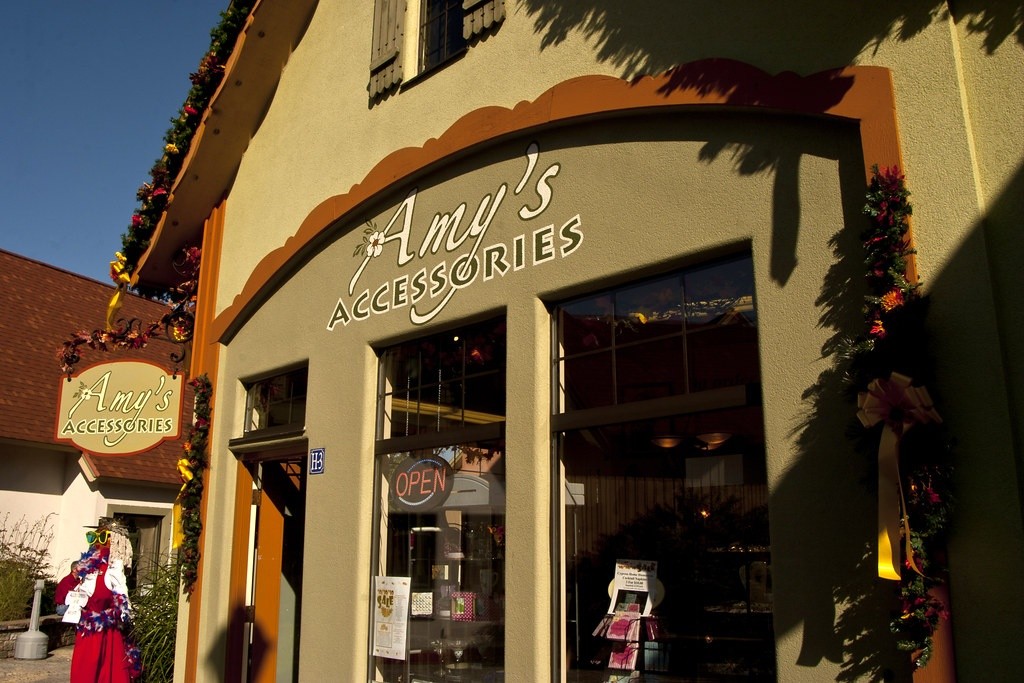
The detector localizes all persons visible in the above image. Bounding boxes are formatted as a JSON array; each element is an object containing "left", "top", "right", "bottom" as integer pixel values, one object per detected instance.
[
  {"left": 70, "top": 516, "right": 133, "bottom": 683},
  {"left": 55, "top": 561, "right": 81, "bottom": 615}
]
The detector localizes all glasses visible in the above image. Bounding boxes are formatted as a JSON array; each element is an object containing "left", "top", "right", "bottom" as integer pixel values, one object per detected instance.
[{"left": 86, "top": 530, "right": 111, "bottom": 544}]
